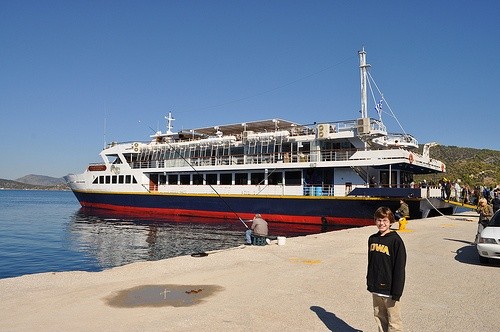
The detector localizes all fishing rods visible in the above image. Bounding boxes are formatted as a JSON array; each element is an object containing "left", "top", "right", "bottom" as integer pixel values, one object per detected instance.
[{"left": 139, "top": 119, "right": 250, "bottom": 230}]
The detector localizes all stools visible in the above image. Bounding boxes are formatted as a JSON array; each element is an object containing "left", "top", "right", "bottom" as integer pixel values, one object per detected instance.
[{"left": 254, "top": 236, "right": 266, "bottom": 246}]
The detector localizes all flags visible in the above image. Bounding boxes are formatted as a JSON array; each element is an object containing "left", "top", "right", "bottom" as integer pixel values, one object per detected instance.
[{"left": 375, "top": 100, "right": 383, "bottom": 113}]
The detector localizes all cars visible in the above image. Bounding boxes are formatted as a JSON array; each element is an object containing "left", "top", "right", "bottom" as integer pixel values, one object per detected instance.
[{"left": 476, "top": 209, "right": 500, "bottom": 263}]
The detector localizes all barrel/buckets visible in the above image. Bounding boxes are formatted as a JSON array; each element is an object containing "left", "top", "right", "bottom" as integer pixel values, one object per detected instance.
[
  {"left": 315, "top": 187, "right": 323, "bottom": 196},
  {"left": 309, "top": 187, "right": 315, "bottom": 196},
  {"left": 277, "top": 236, "right": 286, "bottom": 245}
]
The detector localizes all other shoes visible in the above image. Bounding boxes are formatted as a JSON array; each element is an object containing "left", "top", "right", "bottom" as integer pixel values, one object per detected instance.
[{"left": 244, "top": 242, "right": 251, "bottom": 245}]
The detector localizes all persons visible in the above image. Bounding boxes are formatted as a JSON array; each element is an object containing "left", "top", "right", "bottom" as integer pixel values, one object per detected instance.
[
  {"left": 366, "top": 207, "right": 406, "bottom": 332},
  {"left": 284, "top": 151, "right": 290, "bottom": 163},
  {"left": 290, "top": 129, "right": 299, "bottom": 136},
  {"left": 471, "top": 198, "right": 493, "bottom": 246},
  {"left": 244, "top": 214, "right": 268, "bottom": 245},
  {"left": 455, "top": 180, "right": 461, "bottom": 202},
  {"left": 461, "top": 184, "right": 500, "bottom": 214},
  {"left": 369, "top": 175, "right": 377, "bottom": 197},
  {"left": 409, "top": 179, "right": 454, "bottom": 201},
  {"left": 298, "top": 153, "right": 305, "bottom": 162},
  {"left": 395, "top": 199, "right": 409, "bottom": 221}
]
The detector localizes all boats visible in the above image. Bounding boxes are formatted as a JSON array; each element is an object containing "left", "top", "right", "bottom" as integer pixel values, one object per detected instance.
[{"left": 60, "top": 43, "right": 458, "bottom": 236}]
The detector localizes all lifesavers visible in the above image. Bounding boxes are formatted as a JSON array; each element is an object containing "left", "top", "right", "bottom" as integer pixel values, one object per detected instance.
[
  {"left": 409, "top": 154, "right": 413, "bottom": 162},
  {"left": 442, "top": 164, "right": 444, "bottom": 172}
]
